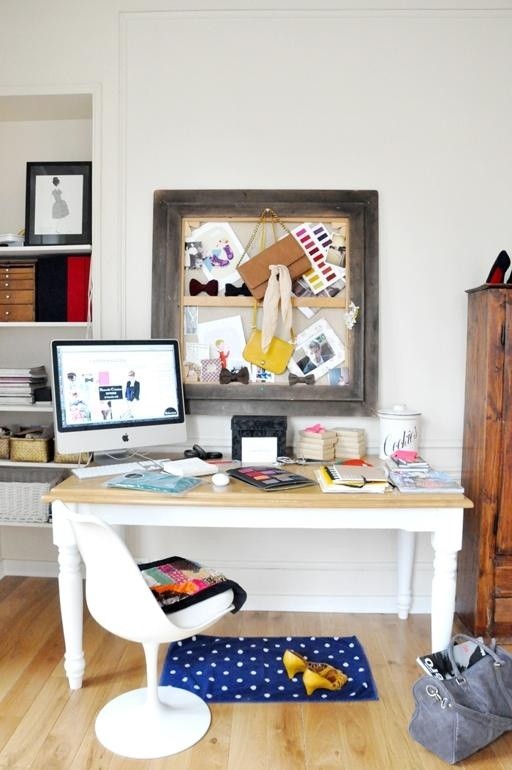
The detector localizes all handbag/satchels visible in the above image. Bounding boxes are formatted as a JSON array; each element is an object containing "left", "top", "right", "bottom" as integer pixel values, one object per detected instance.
[{"left": 407, "top": 632, "right": 512, "bottom": 766}]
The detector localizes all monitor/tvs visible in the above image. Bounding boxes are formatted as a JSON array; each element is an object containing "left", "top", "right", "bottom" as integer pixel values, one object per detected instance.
[{"left": 50, "top": 339, "right": 188, "bottom": 466}]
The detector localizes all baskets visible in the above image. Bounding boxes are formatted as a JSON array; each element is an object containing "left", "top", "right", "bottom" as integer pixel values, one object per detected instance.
[
  {"left": 53, "top": 452, "right": 91, "bottom": 464},
  {"left": 9, "top": 437, "right": 49, "bottom": 463},
  {"left": 0, "top": 467, "right": 66, "bottom": 524},
  {"left": 0, "top": 435, "right": 11, "bottom": 459}
]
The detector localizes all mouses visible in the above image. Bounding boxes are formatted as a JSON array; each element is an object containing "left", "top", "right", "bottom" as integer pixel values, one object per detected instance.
[{"left": 212, "top": 473, "right": 230, "bottom": 486}]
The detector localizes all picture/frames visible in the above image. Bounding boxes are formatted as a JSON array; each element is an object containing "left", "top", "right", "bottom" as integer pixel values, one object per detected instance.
[{"left": 24, "top": 160, "right": 91, "bottom": 244}]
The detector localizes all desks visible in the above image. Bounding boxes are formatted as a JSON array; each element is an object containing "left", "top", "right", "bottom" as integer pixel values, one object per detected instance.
[{"left": 39, "top": 455, "right": 476, "bottom": 687}]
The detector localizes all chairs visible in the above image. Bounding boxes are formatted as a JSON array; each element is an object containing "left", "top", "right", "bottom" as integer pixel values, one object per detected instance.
[{"left": 66, "top": 518, "right": 242, "bottom": 763}]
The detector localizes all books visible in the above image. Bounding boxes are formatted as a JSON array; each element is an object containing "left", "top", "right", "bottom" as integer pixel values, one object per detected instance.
[
  {"left": 105, "top": 467, "right": 202, "bottom": 495},
  {"left": 0, "top": 363, "right": 48, "bottom": 405},
  {"left": 226, "top": 464, "right": 317, "bottom": 493},
  {"left": 298, "top": 427, "right": 466, "bottom": 494},
  {"left": 417, "top": 636, "right": 488, "bottom": 682}
]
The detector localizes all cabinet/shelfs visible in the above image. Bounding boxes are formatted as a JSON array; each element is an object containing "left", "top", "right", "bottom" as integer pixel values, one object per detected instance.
[
  {"left": 457, "top": 283, "right": 512, "bottom": 637},
  {"left": 1, "top": 243, "right": 96, "bottom": 530}
]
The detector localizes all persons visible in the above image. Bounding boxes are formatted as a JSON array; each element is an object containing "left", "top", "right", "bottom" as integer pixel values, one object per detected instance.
[
  {"left": 185, "top": 243, "right": 202, "bottom": 269},
  {"left": 124, "top": 369, "right": 141, "bottom": 418},
  {"left": 303, "top": 340, "right": 333, "bottom": 372},
  {"left": 67, "top": 372, "right": 91, "bottom": 423}
]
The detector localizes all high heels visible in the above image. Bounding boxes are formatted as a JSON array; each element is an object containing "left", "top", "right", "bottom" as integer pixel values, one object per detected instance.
[
  {"left": 282, "top": 648, "right": 314, "bottom": 681},
  {"left": 302, "top": 661, "right": 350, "bottom": 697}
]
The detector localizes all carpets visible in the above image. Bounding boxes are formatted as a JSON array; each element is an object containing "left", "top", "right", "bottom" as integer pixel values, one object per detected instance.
[{"left": 161, "top": 636, "right": 380, "bottom": 704}]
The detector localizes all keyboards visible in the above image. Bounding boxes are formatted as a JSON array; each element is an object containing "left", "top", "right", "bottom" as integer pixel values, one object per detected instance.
[{"left": 71, "top": 458, "right": 173, "bottom": 480}]
